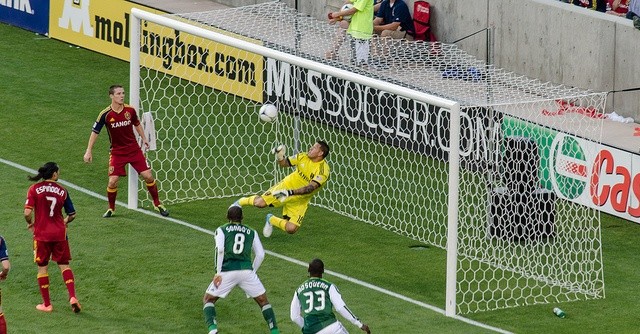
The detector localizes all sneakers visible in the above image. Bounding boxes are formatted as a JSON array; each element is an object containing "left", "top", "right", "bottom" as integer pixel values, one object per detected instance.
[
  {"left": 70, "top": 297, "right": 81, "bottom": 313},
  {"left": 263, "top": 213, "right": 273, "bottom": 237},
  {"left": 103, "top": 209, "right": 116, "bottom": 217},
  {"left": 154, "top": 204, "right": 169, "bottom": 216},
  {"left": 228, "top": 198, "right": 245, "bottom": 210},
  {"left": 36, "top": 304, "right": 53, "bottom": 312}
]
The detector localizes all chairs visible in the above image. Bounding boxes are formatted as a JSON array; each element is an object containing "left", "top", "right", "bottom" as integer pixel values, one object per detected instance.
[{"left": 411, "top": 1, "right": 431, "bottom": 43}]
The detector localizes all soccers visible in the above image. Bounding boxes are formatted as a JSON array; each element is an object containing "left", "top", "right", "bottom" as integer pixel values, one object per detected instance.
[
  {"left": 341, "top": 3, "right": 353, "bottom": 21},
  {"left": 260, "top": 103, "right": 278, "bottom": 122}
]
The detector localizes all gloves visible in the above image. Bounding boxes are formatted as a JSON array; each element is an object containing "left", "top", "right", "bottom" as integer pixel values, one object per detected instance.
[
  {"left": 271, "top": 145, "right": 286, "bottom": 160},
  {"left": 273, "top": 189, "right": 290, "bottom": 203}
]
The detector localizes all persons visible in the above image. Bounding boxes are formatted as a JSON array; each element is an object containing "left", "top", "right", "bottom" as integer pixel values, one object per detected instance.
[
  {"left": 24, "top": 161, "right": 81, "bottom": 313},
  {"left": 373, "top": 0, "right": 416, "bottom": 60},
  {"left": 84, "top": 84, "right": 169, "bottom": 217},
  {"left": 0, "top": 236, "right": 10, "bottom": 334},
  {"left": 290, "top": 258, "right": 370, "bottom": 333},
  {"left": 229, "top": 140, "right": 330, "bottom": 236},
  {"left": 202, "top": 205, "right": 280, "bottom": 333},
  {"left": 326, "top": 0, "right": 384, "bottom": 63},
  {"left": 331, "top": 0, "right": 375, "bottom": 69}
]
